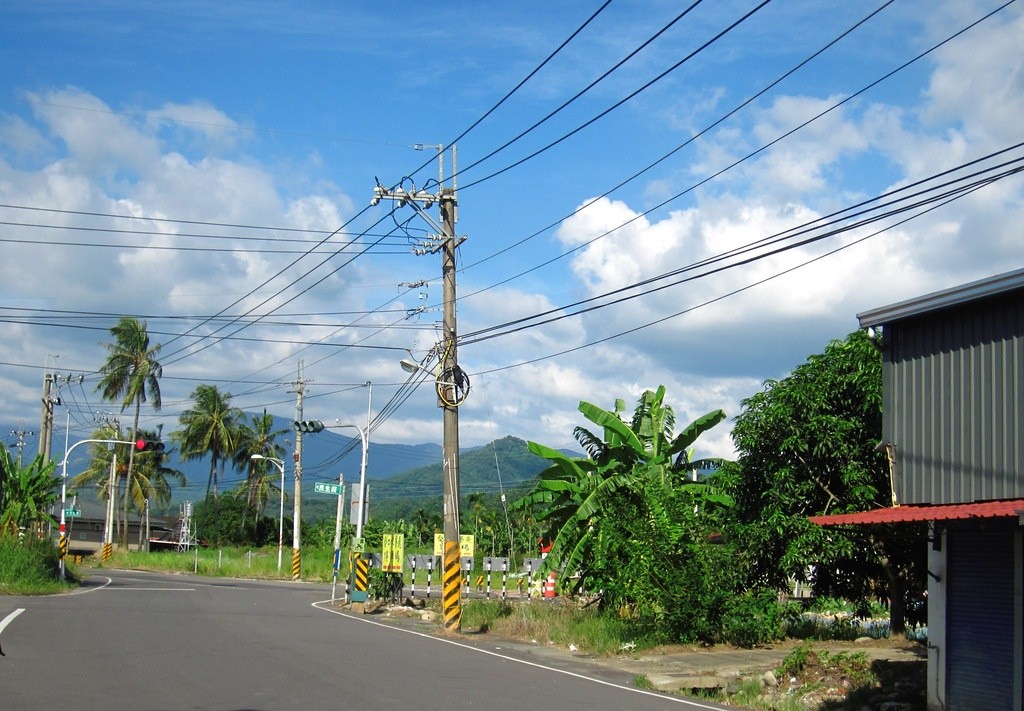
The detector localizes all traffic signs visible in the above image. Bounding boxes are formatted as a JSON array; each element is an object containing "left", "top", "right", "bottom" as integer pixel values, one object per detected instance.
[
  {"left": 315, "top": 482, "right": 342, "bottom": 495},
  {"left": 66, "top": 509, "right": 82, "bottom": 517}
]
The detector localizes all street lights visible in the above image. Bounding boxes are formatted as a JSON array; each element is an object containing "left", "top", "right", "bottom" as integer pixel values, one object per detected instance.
[{"left": 398, "top": 359, "right": 462, "bottom": 635}]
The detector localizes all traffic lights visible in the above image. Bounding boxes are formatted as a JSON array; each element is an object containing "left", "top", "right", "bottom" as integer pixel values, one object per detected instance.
[
  {"left": 292, "top": 419, "right": 324, "bottom": 434},
  {"left": 135, "top": 440, "right": 166, "bottom": 451},
  {"left": 251, "top": 453, "right": 285, "bottom": 578}
]
